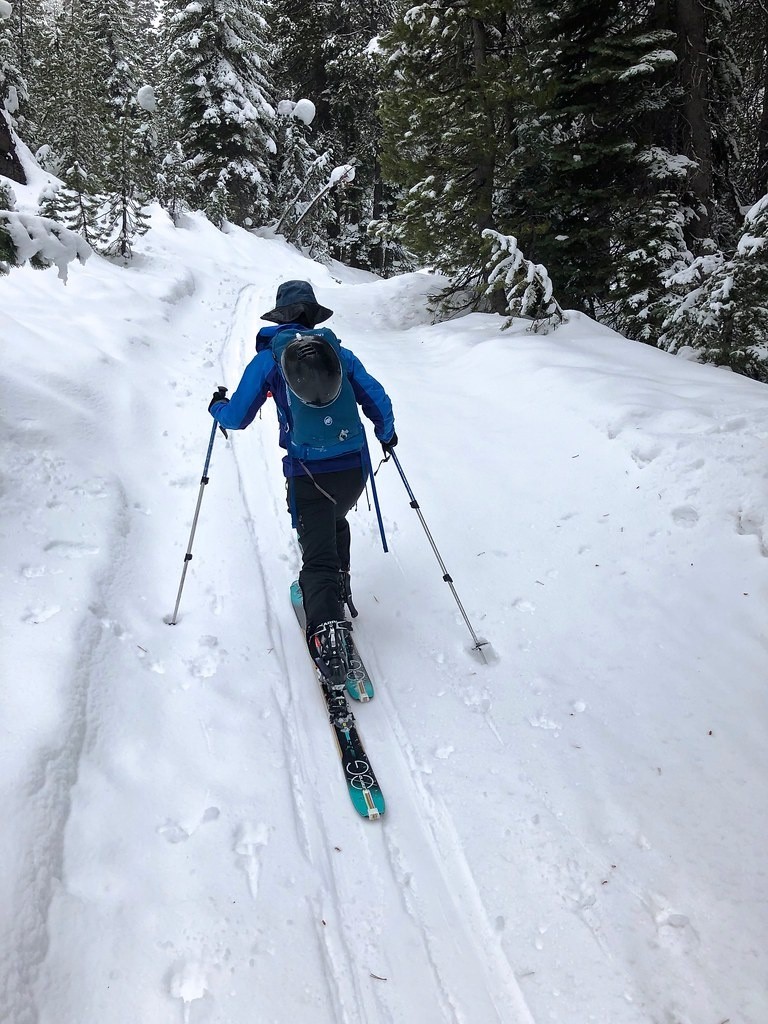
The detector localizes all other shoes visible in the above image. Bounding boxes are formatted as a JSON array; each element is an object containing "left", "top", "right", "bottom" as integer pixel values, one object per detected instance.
[
  {"left": 312, "top": 619, "right": 351, "bottom": 691},
  {"left": 338, "top": 570, "right": 351, "bottom": 604}
]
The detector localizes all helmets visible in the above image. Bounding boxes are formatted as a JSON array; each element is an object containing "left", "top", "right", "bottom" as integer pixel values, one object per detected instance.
[{"left": 280, "top": 333, "right": 342, "bottom": 408}]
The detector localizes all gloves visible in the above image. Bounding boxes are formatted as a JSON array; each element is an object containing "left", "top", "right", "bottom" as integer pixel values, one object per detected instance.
[
  {"left": 208, "top": 392, "right": 229, "bottom": 413},
  {"left": 380, "top": 432, "right": 398, "bottom": 458}
]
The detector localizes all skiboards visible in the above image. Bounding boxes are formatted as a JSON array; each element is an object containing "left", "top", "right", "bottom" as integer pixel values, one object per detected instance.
[{"left": 290, "top": 529, "right": 386, "bottom": 820}]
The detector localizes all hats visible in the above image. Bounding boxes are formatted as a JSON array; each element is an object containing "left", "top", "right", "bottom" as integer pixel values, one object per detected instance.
[{"left": 260, "top": 280, "right": 333, "bottom": 324}]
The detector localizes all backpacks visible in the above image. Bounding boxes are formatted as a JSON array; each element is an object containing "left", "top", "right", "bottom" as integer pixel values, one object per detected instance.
[{"left": 272, "top": 328, "right": 364, "bottom": 461}]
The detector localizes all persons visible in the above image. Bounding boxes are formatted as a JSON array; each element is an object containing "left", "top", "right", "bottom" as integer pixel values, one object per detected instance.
[{"left": 208, "top": 281, "right": 398, "bottom": 692}]
